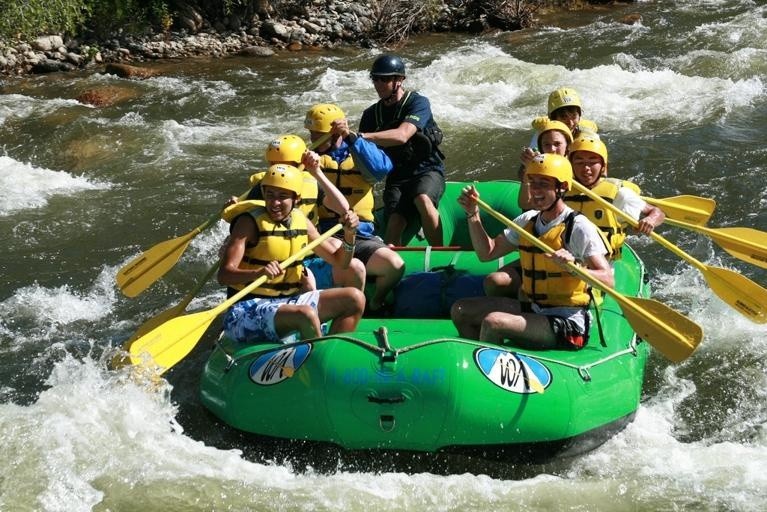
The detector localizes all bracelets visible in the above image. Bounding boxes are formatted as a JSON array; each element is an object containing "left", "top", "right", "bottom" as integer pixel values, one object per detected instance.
[
  {"left": 570, "top": 262, "right": 582, "bottom": 277},
  {"left": 467, "top": 210, "right": 480, "bottom": 219},
  {"left": 342, "top": 240, "right": 358, "bottom": 255}
]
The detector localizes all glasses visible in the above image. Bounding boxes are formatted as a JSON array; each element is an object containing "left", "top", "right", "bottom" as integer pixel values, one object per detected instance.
[{"left": 372, "top": 77, "right": 389, "bottom": 82}]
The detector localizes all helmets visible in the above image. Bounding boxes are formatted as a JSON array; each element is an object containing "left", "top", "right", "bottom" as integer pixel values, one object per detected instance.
[
  {"left": 370, "top": 55, "right": 405, "bottom": 78},
  {"left": 266, "top": 135, "right": 308, "bottom": 163},
  {"left": 537, "top": 121, "right": 572, "bottom": 141},
  {"left": 261, "top": 163, "right": 303, "bottom": 195},
  {"left": 569, "top": 136, "right": 608, "bottom": 165},
  {"left": 305, "top": 105, "right": 346, "bottom": 133},
  {"left": 548, "top": 87, "right": 582, "bottom": 118},
  {"left": 526, "top": 153, "right": 572, "bottom": 192}
]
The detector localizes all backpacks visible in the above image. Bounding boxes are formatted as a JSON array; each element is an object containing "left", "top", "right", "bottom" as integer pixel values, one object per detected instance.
[{"left": 375, "top": 94, "right": 445, "bottom": 162}]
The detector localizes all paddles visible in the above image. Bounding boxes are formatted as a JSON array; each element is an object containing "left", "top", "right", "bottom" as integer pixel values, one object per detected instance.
[
  {"left": 129, "top": 220, "right": 345, "bottom": 378},
  {"left": 471, "top": 196, "right": 702, "bottom": 362},
  {"left": 111, "top": 161, "right": 306, "bottom": 377},
  {"left": 534, "top": 152, "right": 767, "bottom": 324},
  {"left": 641, "top": 197, "right": 716, "bottom": 226},
  {"left": 663, "top": 218, "right": 766, "bottom": 268},
  {"left": 116, "top": 133, "right": 333, "bottom": 298}
]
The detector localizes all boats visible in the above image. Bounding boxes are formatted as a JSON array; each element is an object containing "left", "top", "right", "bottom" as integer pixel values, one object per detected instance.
[{"left": 201, "top": 180, "right": 651, "bottom": 452}]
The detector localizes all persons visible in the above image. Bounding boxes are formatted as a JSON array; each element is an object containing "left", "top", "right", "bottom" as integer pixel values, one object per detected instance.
[
  {"left": 531, "top": 117, "right": 571, "bottom": 158},
  {"left": 450, "top": 155, "right": 615, "bottom": 348},
  {"left": 305, "top": 105, "right": 405, "bottom": 317},
  {"left": 526, "top": 89, "right": 600, "bottom": 147},
  {"left": 483, "top": 136, "right": 666, "bottom": 296},
  {"left": 217, "top": 164, "right": 367, "bottom": 341},
  {"left": 353, "top": 51, "right": 443, "bottom": 247},
  {"left": 222, "top": 134, "right": 367, "bottom": 291}
]
